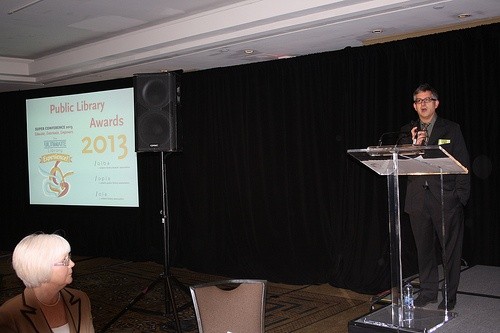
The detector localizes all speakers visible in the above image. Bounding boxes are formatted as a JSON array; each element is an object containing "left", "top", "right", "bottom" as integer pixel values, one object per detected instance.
[{"left": 132, "top": 70, "right": 177, "bottom": 152}]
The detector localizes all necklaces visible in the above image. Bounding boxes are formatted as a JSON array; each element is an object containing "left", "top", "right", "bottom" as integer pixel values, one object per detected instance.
[{"left": 37, "top": 292, "right": 61, "bottom": 306}]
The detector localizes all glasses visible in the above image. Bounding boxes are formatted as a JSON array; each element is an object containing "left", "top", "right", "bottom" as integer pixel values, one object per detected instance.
[
  {"left": 414, "top": 97, "right": 437, "bottom": 104},
  {"left": 53, "top": 253, "right": 71, "bottom": 266}
]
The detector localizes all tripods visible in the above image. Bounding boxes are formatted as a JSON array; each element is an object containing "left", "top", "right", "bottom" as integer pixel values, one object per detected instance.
[{"left": 101, "top": 151, "right": 197, "bottom": 333}]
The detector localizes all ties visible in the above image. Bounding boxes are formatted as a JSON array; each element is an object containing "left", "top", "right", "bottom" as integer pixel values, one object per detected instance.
[{"left": 421, "top": 122, "right": 431, "bottom": 146}]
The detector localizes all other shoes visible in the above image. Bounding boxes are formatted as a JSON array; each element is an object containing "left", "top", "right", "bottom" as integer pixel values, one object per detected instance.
[
  {"left": 438, "top": 298, "right": 457, "bottom": 311},
  {"left": 413, "top": 294, "right": 439, "bottom": 307}
]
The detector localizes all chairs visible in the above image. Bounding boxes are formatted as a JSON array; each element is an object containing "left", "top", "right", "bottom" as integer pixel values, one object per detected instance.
[{"left": 188, "top": 278, "right": 267, "bottom": 333}]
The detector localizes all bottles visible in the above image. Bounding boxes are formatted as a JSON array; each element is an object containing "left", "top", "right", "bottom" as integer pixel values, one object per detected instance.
[{"left": 403, "top": 282, "right": 414, "bottom": 321}]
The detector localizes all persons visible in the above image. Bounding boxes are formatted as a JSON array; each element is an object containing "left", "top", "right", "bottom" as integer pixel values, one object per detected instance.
[
  {"left": 397, "top": 83, "right": 471, "bottom": 311},
  {"left": 0, "top": 233, "right": 94, "bottom": 333}
]
